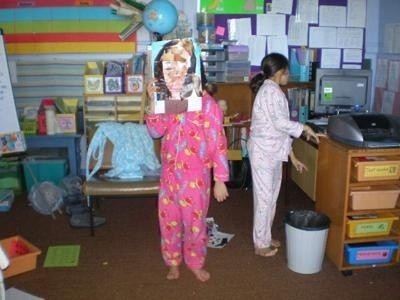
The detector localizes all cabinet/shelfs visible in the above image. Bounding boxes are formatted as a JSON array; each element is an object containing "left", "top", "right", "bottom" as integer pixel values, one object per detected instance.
[
  {"left": 82, "top": 94, "right": 146, "bottom": 132},
  {"left": 289, "top": 136, "right": 316, "bottom": 202},
  {"left": 313, "top": 137, "right": 400, "bottom": 271}
]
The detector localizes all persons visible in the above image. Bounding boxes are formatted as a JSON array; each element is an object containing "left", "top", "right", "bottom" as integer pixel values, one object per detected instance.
[
  {"left": 243, "top": 52, "right": 320, "bottom": 257},
  {"left": 143, "top": 56, "right": 231, "bottom": 282},
  {"left": 153, "top": 40, "right": 202, "bottom": 100}
]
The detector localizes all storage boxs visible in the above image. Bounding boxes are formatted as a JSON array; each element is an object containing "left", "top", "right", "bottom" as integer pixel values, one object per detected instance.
[
  {"left": 22, "top": 156, "right": 68, "bottom": 195},
  {"left": 223, "top": 45, "right": 251, "bottom": 84}
]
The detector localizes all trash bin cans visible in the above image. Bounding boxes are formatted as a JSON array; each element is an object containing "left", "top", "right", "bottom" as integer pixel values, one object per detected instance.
[{"left": 283, "top": 210, "right": 331, "bottom": 275}]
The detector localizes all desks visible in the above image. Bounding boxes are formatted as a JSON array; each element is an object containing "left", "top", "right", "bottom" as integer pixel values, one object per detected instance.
[{"left": 21, "top": 133, "right": 79, "bottom": 177}]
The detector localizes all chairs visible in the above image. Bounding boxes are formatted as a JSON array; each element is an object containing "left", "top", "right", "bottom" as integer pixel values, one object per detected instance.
[{"left": 81, "top": 126, "right": 160, "bottom": 237}]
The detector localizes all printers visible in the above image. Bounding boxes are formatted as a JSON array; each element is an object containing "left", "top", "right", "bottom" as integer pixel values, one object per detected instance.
[{"left": 326, "top": 114, "right": 400, "bottom": 149}]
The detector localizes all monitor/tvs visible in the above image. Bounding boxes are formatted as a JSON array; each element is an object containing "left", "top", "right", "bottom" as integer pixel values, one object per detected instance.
[{"left": 314, "top": 67, "right": 375, "bottom": 115}]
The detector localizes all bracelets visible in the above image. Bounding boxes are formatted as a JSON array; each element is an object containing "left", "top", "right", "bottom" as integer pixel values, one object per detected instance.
[{"left": 145, "top": 106, "right": 155, "bottom": 116}]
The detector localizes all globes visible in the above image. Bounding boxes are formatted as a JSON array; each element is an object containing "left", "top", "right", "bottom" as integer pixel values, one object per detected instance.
[{"left": 142, "top": 0, "right": 178, "bottom": 45}]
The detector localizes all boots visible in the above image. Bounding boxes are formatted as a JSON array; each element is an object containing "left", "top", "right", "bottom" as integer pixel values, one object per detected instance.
[
  {"left": 70, "top": 207, "right": 107, "bottom": 227},
  {"left": 63, "top": 194, "right": 96, "bottom": 218}
]
